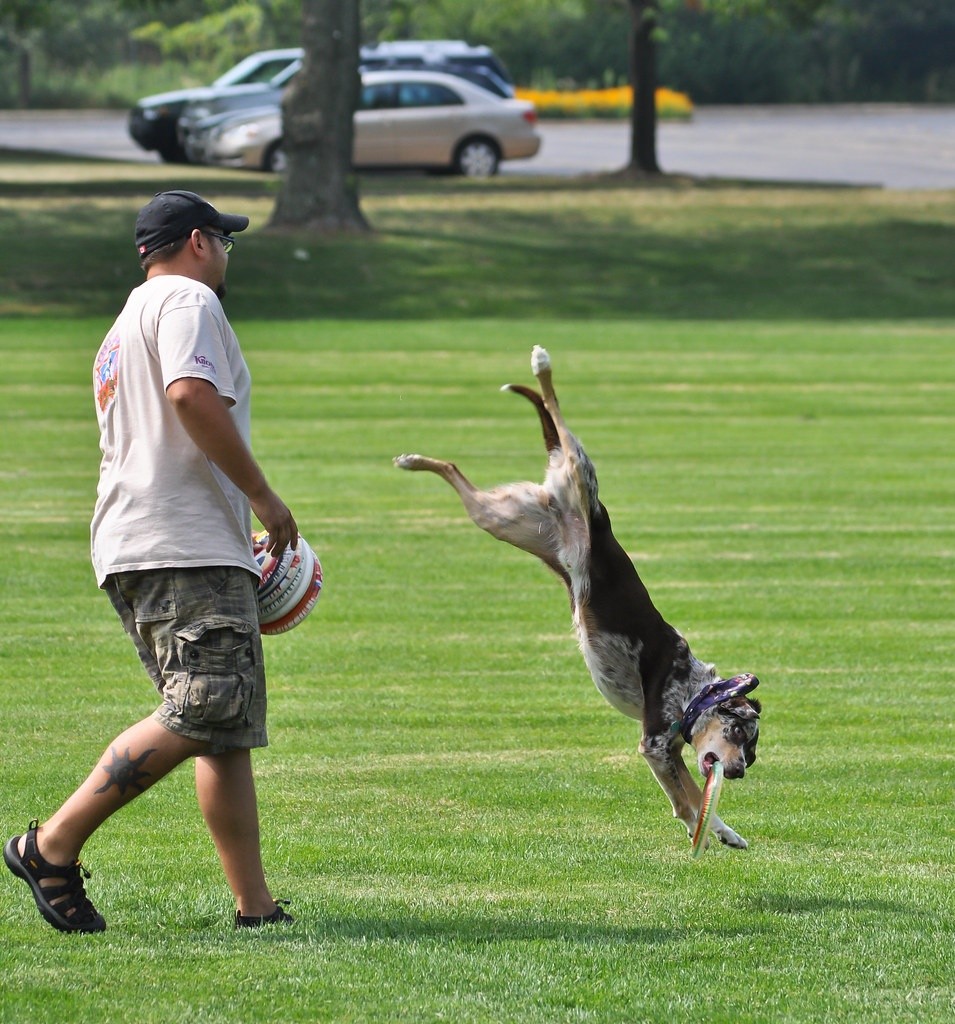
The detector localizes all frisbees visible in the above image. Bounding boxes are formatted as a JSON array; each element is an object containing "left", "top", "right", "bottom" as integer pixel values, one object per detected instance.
[
  {"left": 251, "top": 530, "right": 324, "bottom": 636},
  {"left": 690, "top": 760, "right": 725, "bottom": 859}
]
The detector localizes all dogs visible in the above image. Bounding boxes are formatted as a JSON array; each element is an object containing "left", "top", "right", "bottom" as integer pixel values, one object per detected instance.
[{"left": 395, "top": 344, "right": 762, "bottom": 858}]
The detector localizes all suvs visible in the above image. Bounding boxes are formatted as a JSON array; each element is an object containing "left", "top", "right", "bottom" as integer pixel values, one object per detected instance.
[
  {"left": 128, "top": 38, "right": 511, "bottom": 163},
  {"left": 178, "top": 45, "right": 515, "bottom": 164}
]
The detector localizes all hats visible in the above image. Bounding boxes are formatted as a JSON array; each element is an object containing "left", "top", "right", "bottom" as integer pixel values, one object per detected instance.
[{"left": 135, "top": 189, "right": 250, "bottom": 259}]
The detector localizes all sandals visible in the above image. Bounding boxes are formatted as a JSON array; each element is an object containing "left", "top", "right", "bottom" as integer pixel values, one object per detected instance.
[
  {"left": 3, "top": 818, "right": 108, "bottom": 933},
  {"left": 235, "top": 898, "right": 293, "bottom": 928}
]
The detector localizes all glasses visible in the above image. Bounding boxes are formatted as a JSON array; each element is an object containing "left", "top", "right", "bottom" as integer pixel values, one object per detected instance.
[{"left": 200, "top": 229, "right": 235, "bottom": 252}]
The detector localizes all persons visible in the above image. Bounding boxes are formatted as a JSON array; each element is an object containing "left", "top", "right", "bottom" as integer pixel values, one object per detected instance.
[{"left": 3, "top": 189, "right": 323, "bottom": 937}]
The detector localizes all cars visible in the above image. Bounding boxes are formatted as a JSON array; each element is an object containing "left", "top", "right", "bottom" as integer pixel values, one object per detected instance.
[{"left": 188, "top": 65, "right": 543, "bottom": 177}]
